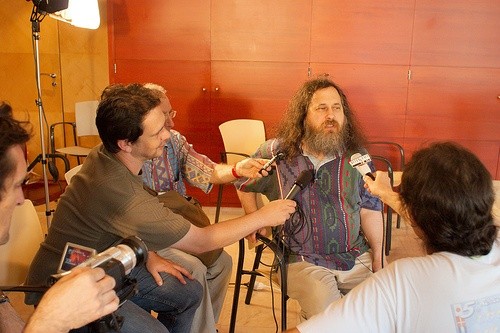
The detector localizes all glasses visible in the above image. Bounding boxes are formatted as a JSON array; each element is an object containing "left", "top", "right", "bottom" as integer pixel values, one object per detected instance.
[{"left": 164, "top": 109, "right": 177, "bottom": 120}]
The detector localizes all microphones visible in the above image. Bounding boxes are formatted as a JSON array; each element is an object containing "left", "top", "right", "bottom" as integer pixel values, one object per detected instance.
[
  {"left": 286, "top": 170, "right": 314, "bottom": 200},
  {"left": 349, "top": 153, "right": 375, "bottom": 182}
]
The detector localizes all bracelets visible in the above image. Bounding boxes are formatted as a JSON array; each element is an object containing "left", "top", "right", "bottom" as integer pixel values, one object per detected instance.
[
  {"left": 232, "top": 163, "right": 240, "bottom": 179},
  {"left": 0, "top": 294, "right": 10, "bottom": 304}
]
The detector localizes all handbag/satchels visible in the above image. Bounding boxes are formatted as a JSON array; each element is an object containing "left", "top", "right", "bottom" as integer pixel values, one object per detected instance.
[{"left": 154, "top": 191, "right": 224, "bottom": 268}]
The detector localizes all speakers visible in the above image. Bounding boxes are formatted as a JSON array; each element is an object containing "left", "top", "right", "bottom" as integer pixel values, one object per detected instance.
[{"left": 32, "top": 0, "right": 68, "bottom": 13}]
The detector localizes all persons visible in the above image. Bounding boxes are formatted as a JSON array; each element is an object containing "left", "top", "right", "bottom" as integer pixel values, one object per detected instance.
[
  {"left": 138, "top": 81, "right": 276, "bottom": 333},
  {"left": 22, "top": 83, "right": 297, "bottom": 333},
  {"left": 236, "top": 77, "right": 388, "bottom": 323},
  {"left": 0, "top": 99, "right": 120, "bottom": 332},
  {"left": 279, "top": 139, "right": 500, "bottom": 333}
]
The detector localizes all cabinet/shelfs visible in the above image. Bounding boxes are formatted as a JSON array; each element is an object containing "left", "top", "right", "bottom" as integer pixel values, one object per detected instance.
[{"left": 107, "top": 0, "right": 500, "bottom": 207}]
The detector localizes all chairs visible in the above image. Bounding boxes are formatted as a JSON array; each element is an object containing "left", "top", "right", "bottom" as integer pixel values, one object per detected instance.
[
  {"left": 215, "top": 119, "right": 266, "bottom": 223},
  {"left": 0, "top": 199, "right": 54, "bottom": 333},
  {"left": 49, "top": 121, "right": 94, "bottom": 186},
  {"left": 229, "top": 193, "right": 287, "bottom": 333},
  {"left": 363, "top": 142, "right": 405, "bottom": 255}
]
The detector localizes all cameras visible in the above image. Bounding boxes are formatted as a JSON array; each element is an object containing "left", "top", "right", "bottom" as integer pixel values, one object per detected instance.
[{"left": 48, "top": 235, "right": 149, "bottom": 333}]
